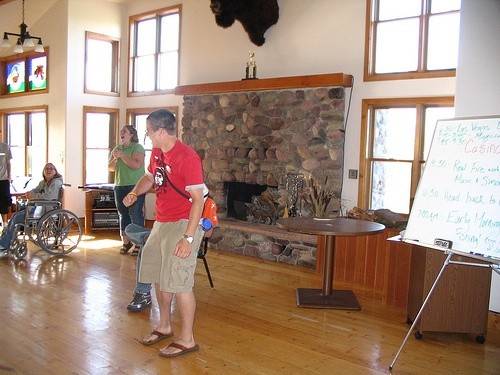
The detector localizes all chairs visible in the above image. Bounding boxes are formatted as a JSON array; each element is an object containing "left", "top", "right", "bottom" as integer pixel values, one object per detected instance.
[{"left": 198, "top": 225, "right": 218, "bottom": 289}]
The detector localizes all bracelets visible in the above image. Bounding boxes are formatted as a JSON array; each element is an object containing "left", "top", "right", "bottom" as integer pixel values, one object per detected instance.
[
  {"left": 127, "top": 191, "right": 139, "bottom": 197},
  {"left": 119, "top": 154, "right": 124, "bottom": 159}
]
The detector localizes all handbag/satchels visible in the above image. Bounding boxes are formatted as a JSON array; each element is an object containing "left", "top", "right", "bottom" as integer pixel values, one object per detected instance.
[{"left": 188, "top": 180, "right": 210, "bottom": 206}]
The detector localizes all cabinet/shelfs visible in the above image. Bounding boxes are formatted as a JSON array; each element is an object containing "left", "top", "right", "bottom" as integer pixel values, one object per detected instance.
[{"left": 85, "top": 188, "right": 120, "bottom": 233}]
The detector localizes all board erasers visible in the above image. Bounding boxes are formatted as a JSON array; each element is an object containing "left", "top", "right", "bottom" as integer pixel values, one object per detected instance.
[{"left": 434, "top": 238, "right": 453, "bottom": 249}]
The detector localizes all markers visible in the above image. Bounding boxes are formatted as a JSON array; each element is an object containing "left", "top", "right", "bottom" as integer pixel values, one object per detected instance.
[
  {"left": 470, "top": 251, "right": 484, "bottom": 256},
  {"left": 398, "top": 238, "right": 419, "bottom": 241},
  {"left": 488, "top": 256, "right": 500, "bottom": 261}
]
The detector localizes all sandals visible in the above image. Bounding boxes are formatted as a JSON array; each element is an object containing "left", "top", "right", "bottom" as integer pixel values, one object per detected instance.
[
  {"left": 131, "top": 245, "right": 140, "bottom": 255},
  {"left": 120, "top": 241, "right": 133, "bottom": 253}
]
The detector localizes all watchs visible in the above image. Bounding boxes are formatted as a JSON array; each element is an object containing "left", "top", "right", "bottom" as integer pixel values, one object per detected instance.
[{"left": 182, "top": 234, "right": 194, "bottom": 245}]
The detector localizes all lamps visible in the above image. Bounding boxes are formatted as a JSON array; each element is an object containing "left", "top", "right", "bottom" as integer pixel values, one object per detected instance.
[{"left": 0, "top": 0, "right": 45, "bottom": 53}]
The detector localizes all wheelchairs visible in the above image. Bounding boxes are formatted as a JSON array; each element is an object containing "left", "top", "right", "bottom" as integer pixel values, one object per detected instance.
[{"left": 1, "top": 182, "right": 83, "bottom": 263}]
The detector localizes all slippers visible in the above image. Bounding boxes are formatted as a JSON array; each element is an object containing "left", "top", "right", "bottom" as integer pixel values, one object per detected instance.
[
  {"left": 142, "top": 330, "right": 174, "bottom": 345},
  {"left": 158, "top": 342, "right": 200, "bottom": 358}
]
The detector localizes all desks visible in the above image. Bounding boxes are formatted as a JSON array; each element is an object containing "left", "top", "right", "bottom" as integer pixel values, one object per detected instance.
[{"left": 275, "top": 216, "right": 386, "bottom": 310}]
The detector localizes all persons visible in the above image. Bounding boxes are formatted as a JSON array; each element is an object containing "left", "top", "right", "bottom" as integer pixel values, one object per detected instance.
[
  {"left": 0, "top": 163, "right": 64, "bottom": 259},
  {"left": 0, "top": 132, "right": 13, "bottom": 228},
  {"left": 124, "top": 197, "right": 219, "bottom": 313},
  {"left": 106, "top": 123, "right": 147, "bottom": 257},
  {"left": 122, "top": 108, "right": 206, "bottom": 358}
]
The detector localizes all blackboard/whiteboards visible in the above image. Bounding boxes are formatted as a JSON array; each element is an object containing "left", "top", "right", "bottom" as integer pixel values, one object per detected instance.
[{"left": 387, "top": 114, "right": 500, "bottom": 267}]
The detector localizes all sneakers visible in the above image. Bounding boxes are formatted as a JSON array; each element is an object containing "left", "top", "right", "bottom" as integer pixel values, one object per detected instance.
[{"left": 126, "top": 291, "right": 152, "bottom": 311}]
